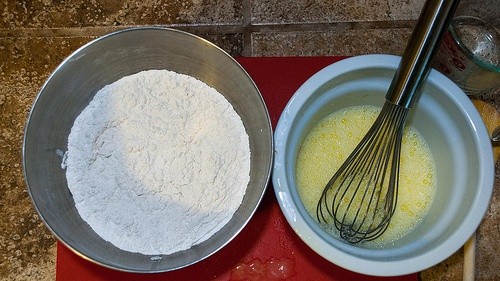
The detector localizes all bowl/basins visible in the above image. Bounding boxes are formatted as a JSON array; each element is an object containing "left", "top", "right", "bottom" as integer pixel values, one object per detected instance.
[
  {"left": 21, "top": 25, "right": 276, "bottom": 274},
  {"left": 269, "top": 51, "right": 495, "bottom": 278}
]
